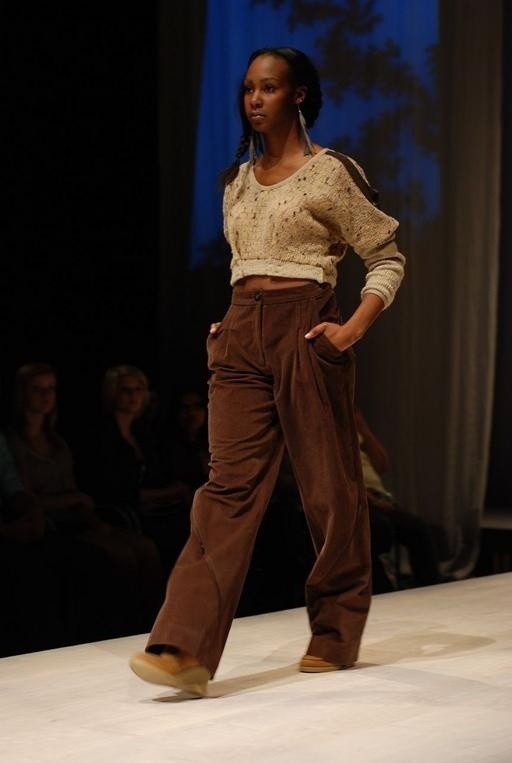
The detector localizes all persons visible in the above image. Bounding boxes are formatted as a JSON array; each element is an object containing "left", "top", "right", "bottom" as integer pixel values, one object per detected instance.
[
  {"left": 134, "top": 48, "right": 403, "bottom": 694},
  {"left": 0, "top": 363, "right": 434, "bottom": 598}
]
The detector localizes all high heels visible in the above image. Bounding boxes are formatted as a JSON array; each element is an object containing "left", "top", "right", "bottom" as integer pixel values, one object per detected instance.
[
  {"left": 300, "top": 655, "right": 341, "bottom": 673},
  {"left": 129, "top": 650, "right": 209, "bottom": 697}
]
